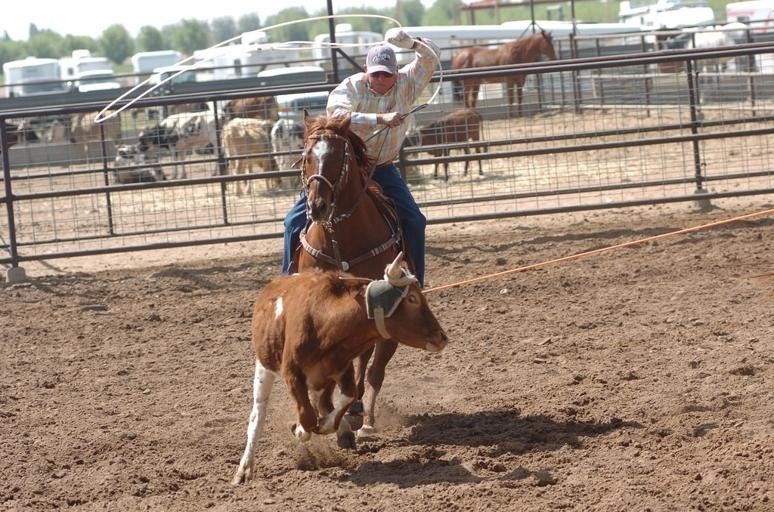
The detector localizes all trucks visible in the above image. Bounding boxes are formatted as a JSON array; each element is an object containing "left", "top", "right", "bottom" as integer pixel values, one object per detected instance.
[{"left": 3, "top": 0, "right": 773, "bottom": 127}]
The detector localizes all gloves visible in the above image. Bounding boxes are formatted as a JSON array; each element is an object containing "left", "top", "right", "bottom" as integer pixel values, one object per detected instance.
[{"left": 387, "top": 29, "right": 414, "bottom": 49}]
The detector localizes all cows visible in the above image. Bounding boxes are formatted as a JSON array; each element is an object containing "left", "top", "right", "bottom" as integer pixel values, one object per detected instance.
[
  {"left": 4, "top": 121, "right": 39, "bottom": 150},
  {"left": 404, "top": 109, "right": 489, "bottom": 182},
  {"left": 230, "top": 251, "right": 449, "bottom": 485},
  {"left": 68, "top": 95, "right": 306, "bottom": 197}
]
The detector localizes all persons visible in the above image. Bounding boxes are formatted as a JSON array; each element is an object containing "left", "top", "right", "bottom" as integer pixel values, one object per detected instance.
[{"left": 281, "top": 25, "right": 441, "bottom": 287}]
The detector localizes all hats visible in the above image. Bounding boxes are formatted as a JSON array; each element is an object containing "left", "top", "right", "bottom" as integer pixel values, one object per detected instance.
[{"left": 366, "top": 45, "right": 398, "bottom": 74}]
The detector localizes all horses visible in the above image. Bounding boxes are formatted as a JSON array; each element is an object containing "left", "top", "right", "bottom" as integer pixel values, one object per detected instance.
[
  {"left": 451, "top": 29, "right": 557, "bottom": 122},
  {"left": 296, "top": 108, "right": 417, "bottom": 438}
]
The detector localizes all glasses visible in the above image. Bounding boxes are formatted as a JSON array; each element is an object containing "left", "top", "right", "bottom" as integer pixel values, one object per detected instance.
[{"left": 370, "top": 72, "right": 393, "bottom": 78}]
